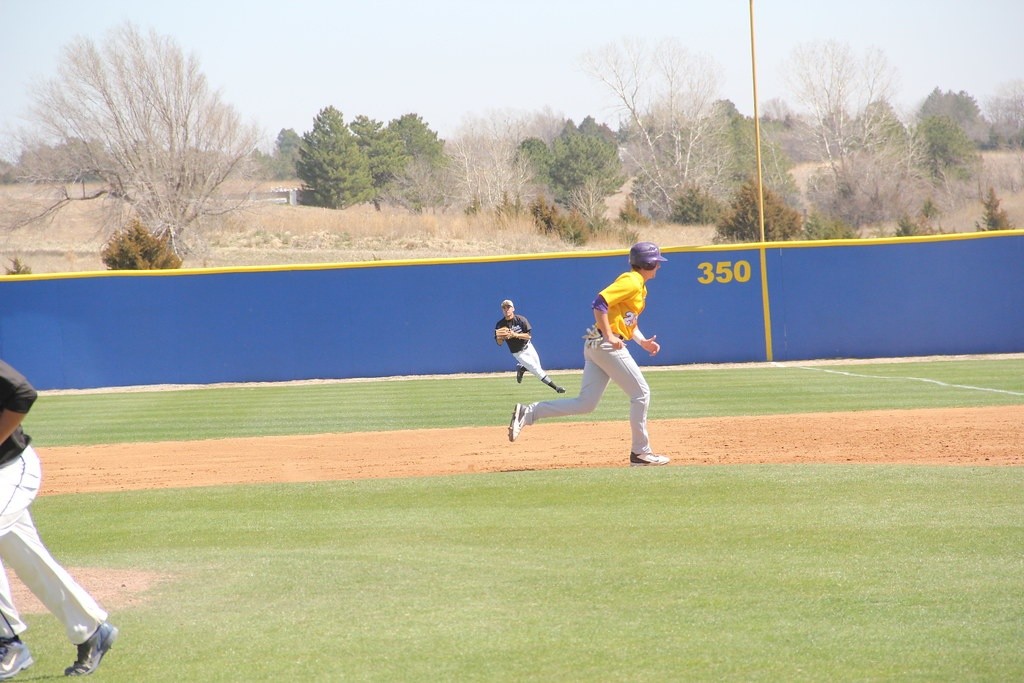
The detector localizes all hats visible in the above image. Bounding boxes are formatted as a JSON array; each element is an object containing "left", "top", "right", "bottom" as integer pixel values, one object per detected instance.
[{"left": 501, "top": 300, "right": 515, "bottom": 308}]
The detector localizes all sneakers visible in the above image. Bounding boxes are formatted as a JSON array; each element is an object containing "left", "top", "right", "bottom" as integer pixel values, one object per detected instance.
[
  {"left": 1, "top": 636, "right": 34, "bottom": 681},
  {"left": 507, "top": 403, "right": 528, "bottom": 441},
  {"left": 630, "top": 452, "right": 671, "bottom": 466},
  {"left": 63, "top": 625, "right": 120, "bottom": 677}
]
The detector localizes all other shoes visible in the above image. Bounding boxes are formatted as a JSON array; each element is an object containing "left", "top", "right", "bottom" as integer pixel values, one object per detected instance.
[
  {"left": 556, "top": 386, "right": 566, "bottom": 394},
  {"left": 516, "top": 363, "right": 525, "bottom": 384}
]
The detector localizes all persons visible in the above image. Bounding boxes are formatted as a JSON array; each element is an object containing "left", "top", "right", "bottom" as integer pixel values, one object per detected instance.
[
  {"left": 494, "top": 300, "right": 565, "bottom": 394},
  {"left": 508, "top": 242, "right": 670, "bottom": 466},
  {"left": 0, "top": 358, "right": 118, "bottom": 678}
]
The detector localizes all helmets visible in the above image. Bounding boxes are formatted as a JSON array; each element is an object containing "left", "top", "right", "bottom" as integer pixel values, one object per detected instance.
[{"left": 629, "top": 242, "right": 669, "bottom": 269}]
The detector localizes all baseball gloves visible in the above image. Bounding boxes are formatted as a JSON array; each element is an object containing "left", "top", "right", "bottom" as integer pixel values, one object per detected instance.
[{"left": 496, "top": 326, "right": 513, "bottom": 340}]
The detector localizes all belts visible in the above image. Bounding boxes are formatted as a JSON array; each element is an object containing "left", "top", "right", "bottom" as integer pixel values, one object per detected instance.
[{"left": 597, "top": 329, "right": 624, "bottom": 341}]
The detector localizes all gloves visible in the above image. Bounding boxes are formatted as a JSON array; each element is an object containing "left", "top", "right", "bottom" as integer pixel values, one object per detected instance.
[{"left": 584, "top": 325, "right": 604, "bottom": 350}]
